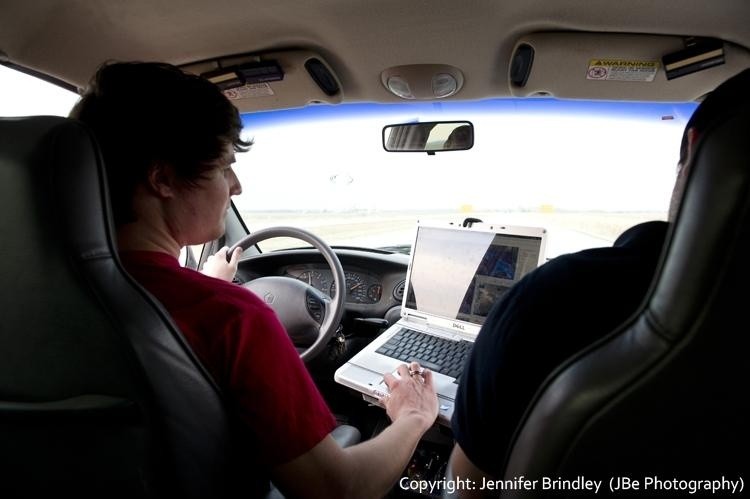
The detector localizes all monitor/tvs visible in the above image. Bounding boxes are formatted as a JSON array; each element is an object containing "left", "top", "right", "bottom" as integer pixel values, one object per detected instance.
[{"left": 333, "top": 219, "right": 548, "bottom": 425}]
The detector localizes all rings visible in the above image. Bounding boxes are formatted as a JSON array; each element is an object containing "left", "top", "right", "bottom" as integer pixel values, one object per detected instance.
[{"left": 410, "top": 368, "right": 423, "bottom": 378}]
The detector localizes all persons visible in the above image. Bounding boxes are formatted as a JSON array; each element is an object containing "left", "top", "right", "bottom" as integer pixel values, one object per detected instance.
[
  {"left": 67, "top": 58, "right": 439, "bottom": 499},
  {"left": 443, "top": 66, "right": 748, "bottom": 497}
]
